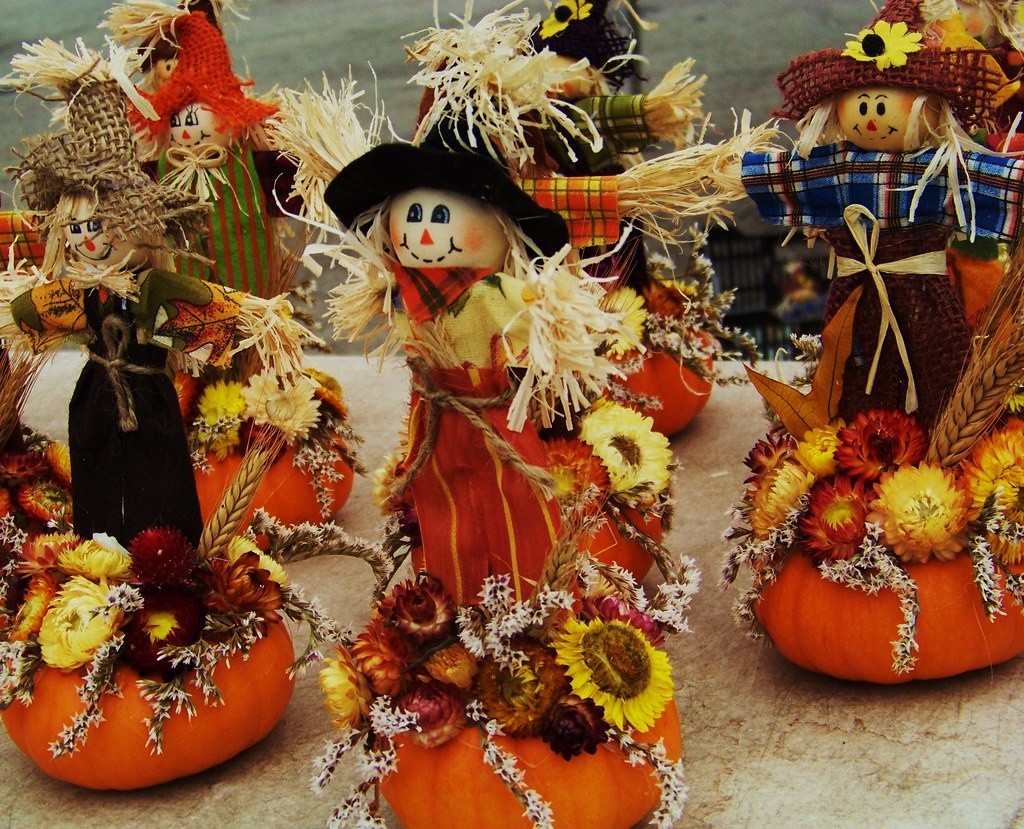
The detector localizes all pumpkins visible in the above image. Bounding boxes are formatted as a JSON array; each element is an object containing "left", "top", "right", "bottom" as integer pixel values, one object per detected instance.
[{"left": 0, "top": 56, "right": 1024, "bottom": 829}]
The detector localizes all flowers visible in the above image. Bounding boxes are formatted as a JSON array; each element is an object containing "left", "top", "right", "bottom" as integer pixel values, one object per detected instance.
[{"left": 0, "top": 347, "right": 1024, "bottom": 829}]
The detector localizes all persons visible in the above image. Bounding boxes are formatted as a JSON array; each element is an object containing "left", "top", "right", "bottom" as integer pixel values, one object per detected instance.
[
  {"left": 0, "top": 0, "right": 328, "bottom": 551},
  {"left": 708, "top": 0, "right": 1024, "bottom": 433},
  {"left": 267, "top": 0, "right": 721, "bottom": 611}
]
